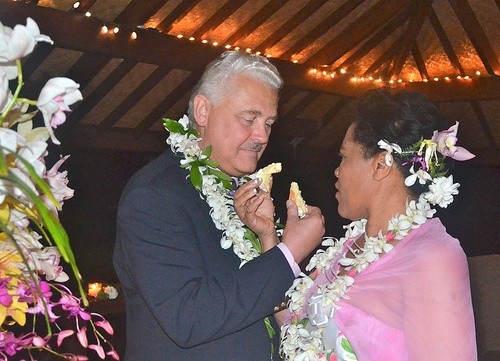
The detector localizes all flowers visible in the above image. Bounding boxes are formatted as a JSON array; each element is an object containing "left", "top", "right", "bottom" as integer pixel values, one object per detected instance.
[
  {"left": 376, "top": 122, "right": 475, "bottom": 187},
  {"left": 1, "top": 15, "right": 124, "bottom": 360}
]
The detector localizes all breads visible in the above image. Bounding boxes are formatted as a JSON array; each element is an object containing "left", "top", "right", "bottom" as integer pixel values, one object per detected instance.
[
  {"left": 249, "top": 162, "right": 282, "bottom": 191},
  {"left": 288, "top": 182, "right": 308, "bottom": 219}
]
